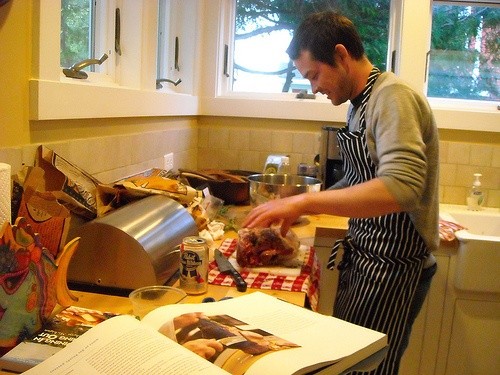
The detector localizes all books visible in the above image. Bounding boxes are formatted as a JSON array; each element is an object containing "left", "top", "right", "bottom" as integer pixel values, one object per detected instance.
[{"left": 19, "top": 290, "right": 387, "bottom": 375}]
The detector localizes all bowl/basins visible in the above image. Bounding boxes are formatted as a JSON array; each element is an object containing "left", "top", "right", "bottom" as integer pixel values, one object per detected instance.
[
  {"left": 186, "top": 169, "right": 262, "bottom": 202},
  {"left": 128, "top": 285, "right": 188, "bottom": 321},
  {"left": 249, "top": 173, "right": 322, "bottom": 224}
]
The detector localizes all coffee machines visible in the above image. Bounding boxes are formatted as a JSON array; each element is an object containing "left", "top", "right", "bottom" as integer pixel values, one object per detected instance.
[{"left": 320, "top": 127, "right": 346, "bottom": 192}]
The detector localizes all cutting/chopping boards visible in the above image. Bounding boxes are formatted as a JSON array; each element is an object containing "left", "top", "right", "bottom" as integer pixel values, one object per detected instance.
[{"left": 228, "top": 244, "right": 306, "bottom": 277}]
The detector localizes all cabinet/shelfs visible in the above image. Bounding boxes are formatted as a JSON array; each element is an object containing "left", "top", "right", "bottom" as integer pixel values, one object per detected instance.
[{"left": 315, "top": 246, "right": 451, "bottom": 375}]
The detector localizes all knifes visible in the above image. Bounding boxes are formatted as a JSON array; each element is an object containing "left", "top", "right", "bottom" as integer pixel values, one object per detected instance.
[{"left": 214, "top": 248, "right": 247, "bottom": 290}]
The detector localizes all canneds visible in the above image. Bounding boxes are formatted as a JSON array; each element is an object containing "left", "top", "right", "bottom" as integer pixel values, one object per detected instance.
[{"left": 180, "top": 236, "right": 209, "bottom": 295}]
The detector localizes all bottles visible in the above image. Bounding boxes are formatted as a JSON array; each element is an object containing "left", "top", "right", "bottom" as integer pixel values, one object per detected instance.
[{"left": 298, "top": 163, "right": 317, "bottom": 177}]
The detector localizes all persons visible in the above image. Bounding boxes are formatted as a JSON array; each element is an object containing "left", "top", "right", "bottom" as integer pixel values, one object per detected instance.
[
  {"left": 157, "top": 312, "right": 223, "bottom": 361},
  {"left": 242, "top": 11, "right": 441, "bottom": 375}
]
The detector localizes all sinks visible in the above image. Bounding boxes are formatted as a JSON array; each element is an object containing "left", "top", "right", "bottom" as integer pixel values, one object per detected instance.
[{"left": 438, "top": 203, "right": 500, "bottom": 293}]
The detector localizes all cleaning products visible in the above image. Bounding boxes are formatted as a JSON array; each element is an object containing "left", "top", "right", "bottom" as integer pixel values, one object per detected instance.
[{"left": 465, "top": 173, "right": 484, "bottom": 211}]
